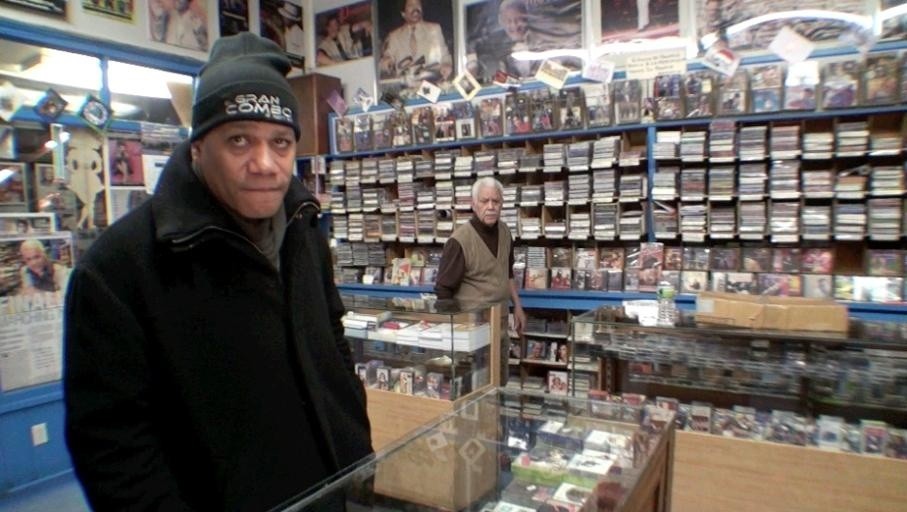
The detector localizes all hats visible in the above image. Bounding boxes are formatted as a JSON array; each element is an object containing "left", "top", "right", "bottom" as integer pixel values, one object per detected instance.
[{"left": 189, "top": 30, "right": 302, "bottom": 144}]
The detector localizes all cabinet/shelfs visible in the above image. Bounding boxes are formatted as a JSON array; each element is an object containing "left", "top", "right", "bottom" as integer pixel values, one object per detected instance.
[{"left": 276, "top": 42, "right": 907, "bottom": 512}]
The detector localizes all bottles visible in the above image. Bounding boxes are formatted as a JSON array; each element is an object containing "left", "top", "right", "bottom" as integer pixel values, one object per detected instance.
[{"left": 656, "top": 289, "right": 677, "bottom": 329}]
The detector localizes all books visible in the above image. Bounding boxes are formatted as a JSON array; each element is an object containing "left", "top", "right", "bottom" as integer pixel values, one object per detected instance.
[
  {"left": 312, "top": 69, "right": 496, "bottom": 401},
  {"left": 498, "top": 49, "right": 907, "bottom": 511}
]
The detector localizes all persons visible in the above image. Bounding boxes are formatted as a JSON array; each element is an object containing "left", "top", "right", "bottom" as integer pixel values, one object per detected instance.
[
  {"left": 471, "top": 0, "right": 559, "bottom": 82},
  {"left": 58, "top": 29, "right": 377, "bottom": 512},
  {"left": 314, "top": 13, "right": 372, "bottom": 68},
  {"left": 0, "top": 126, "right": 133, "bottom": 311},
  {"left": 262, "top": 2, "right": 302, "bottom": 55},
  {"left": 695, "top": 1, "right": 733, "bottom": 59},
  {"left": 381, "top": 0, "right": 451, "bottom": 82},
  {"left": 147, "top": 1, "right": 209, "bottom": 54},
  {"left": 435, "top": 177, "right": 526, "bottom": 473}
]
